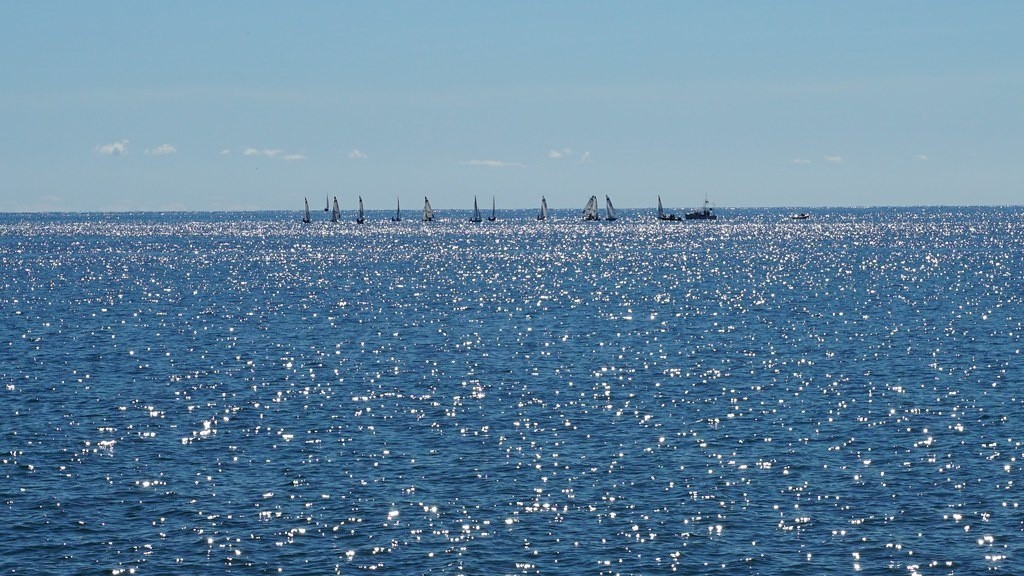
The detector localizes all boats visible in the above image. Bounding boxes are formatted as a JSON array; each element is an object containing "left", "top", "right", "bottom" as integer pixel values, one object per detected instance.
[{"left": 792, "top": 212, "right": 809, "bottom": 219}]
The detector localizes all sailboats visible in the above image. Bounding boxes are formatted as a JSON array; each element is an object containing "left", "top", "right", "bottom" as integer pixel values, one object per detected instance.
[
  {"left": 655, "top": 194, "right": 683, "bottom": 222},
  {"left": 356, "top": 196, "right": 366, "bottom": 225},
  {"left": 324, "top": 193, "right": 331, "bottom": 212},
  {"left": 330, "top": 196, "right": 341, "bottom": 223},
  {"left": 391, "top": 198, "right": 402, "bottom": 222},
  {"left": 537, "top": 196, "right": 550, "bottom": 221},
  {"left": 685, "top": 191, "right": 718, "bottom": 220},
  {"left": 422, "top": 196, "right": 434, "bottom": 222},
  {"left": 302, "top": 197, "right": 313, "bottom": 223},
  {"left": 487, "top": 195, "right": 496, "bottom": 221},
  {"left": 604, "top": 194, "right": 618, "bottom": 222},
  {"left": 583, "top": 195, "right": 601, "bottom": 222},
  {"left": 469, "top": 194, "right": 483, "bottom": 222}
]
